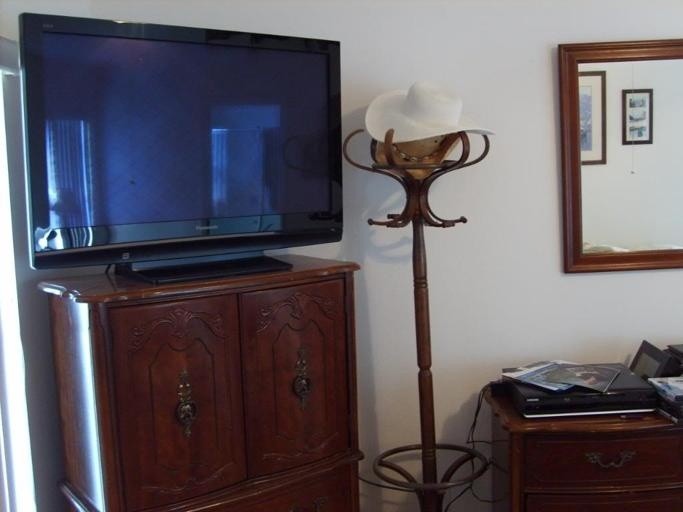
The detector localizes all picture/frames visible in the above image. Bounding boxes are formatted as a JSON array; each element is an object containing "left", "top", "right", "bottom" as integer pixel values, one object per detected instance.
[
  {"left": 621, "top": 89, "right": 653, "bottom": 144},
  {"left": 577, "top": 71, "right": 607, "bottom": 165}
]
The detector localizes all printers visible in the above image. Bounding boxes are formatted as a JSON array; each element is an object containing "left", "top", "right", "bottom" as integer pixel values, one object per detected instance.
[{"left": 501, "top": 363, "right": 658, "bottom": 419}]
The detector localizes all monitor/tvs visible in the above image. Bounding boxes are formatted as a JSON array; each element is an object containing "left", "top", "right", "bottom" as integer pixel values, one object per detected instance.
[{"left": 20, "top": 13, "right": 342, "bottom": 287}]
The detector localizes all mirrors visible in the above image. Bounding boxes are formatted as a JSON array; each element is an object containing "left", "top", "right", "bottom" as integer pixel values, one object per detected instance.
[{"left": 558, "top": 38, "right": 682, "bottom": 273}]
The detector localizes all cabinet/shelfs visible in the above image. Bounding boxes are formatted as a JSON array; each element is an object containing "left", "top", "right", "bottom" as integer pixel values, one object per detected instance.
[
  {"left": 38, "top": 254, "right": 365, "bottom": 512},
  {"left": 483, "top": 387, "right": 682, "bottom": 511}
]
